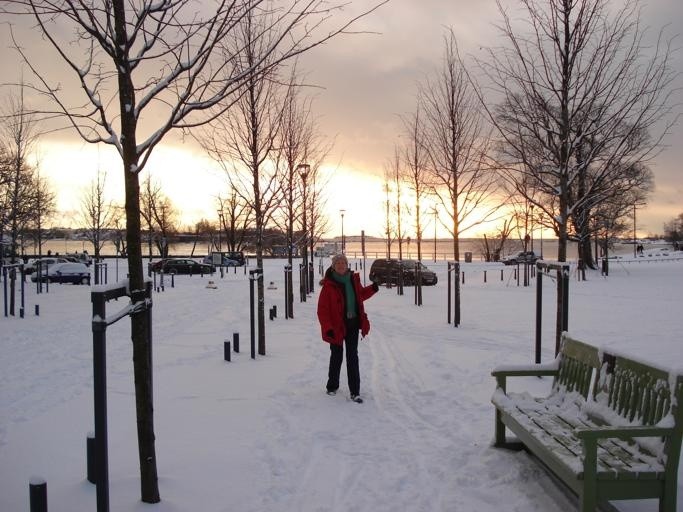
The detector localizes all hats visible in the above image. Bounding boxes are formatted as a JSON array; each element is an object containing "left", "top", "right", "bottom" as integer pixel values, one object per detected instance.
[{"left": 332, "top": 254, "right": 348, "bottom": 269}]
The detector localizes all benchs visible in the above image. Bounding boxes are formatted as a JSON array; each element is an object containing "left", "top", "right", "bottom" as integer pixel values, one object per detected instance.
[{"left": 489, "top": 331, "right": 682, "bottom": 512}]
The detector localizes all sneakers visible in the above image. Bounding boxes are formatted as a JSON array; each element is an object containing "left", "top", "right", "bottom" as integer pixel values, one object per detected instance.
[
  {"left": 351, "top": 393, "right": 362, "bottom": 403},
  {"left": 327, "top": 390, "right": 335, "bottom": 396}
]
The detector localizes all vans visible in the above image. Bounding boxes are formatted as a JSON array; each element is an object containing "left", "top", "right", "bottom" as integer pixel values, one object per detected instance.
[{"left": 370, "top": 257, "right": 437, "bottom": 288}]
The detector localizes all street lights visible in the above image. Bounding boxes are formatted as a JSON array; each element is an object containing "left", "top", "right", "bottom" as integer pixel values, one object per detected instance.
[
  {"left": 527, "top": 196, "right": 536, "bottom": 279},
  {"left": 217, "top": 209, "right": 224, "bottom": 253},
  {"left": 339, "top": 206, "right": 346, "bottom": 258},
  {"left": 296, "top": 147, "right": 312, "bottom": 298},
  {"left": 537, "top": 211, "right": 544, "bottom": 260}
]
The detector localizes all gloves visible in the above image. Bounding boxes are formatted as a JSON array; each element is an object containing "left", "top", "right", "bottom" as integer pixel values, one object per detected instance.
[
  {"left": 327, "top": 331, "right": 333, "bottom": 338},
  {"left": 372, "top": 282, "right": 379, "bottom": 292}
]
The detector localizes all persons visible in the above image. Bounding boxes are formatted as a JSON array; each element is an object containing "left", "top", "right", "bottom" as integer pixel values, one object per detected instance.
[
  {"left": 316, "top": 253, "right": 379, "bottom": 404},
  {"left": 675, "top": 239, "right": 679, "bottom": 250},
  {"left": 637, "top": 245, "right": 639, "bottom": 252},
  {"left": 639, "top": 244, "right": 642, "bottom": 253},
  {"left": 672, "top": 240, "right": 674, "bottom": 247}
]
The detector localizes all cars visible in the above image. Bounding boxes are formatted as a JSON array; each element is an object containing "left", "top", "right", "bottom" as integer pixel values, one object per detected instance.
[
  {"left": 25, "top": 252, "right": 93, "bottom": 286},
  {"left": 147, "top": 250, "right": 244, "bottom": 277}
]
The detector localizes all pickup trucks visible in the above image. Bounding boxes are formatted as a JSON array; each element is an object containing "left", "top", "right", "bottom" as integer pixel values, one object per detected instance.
[{"left": 500, "top": 250, "right": 543, "bottom": 267}]
